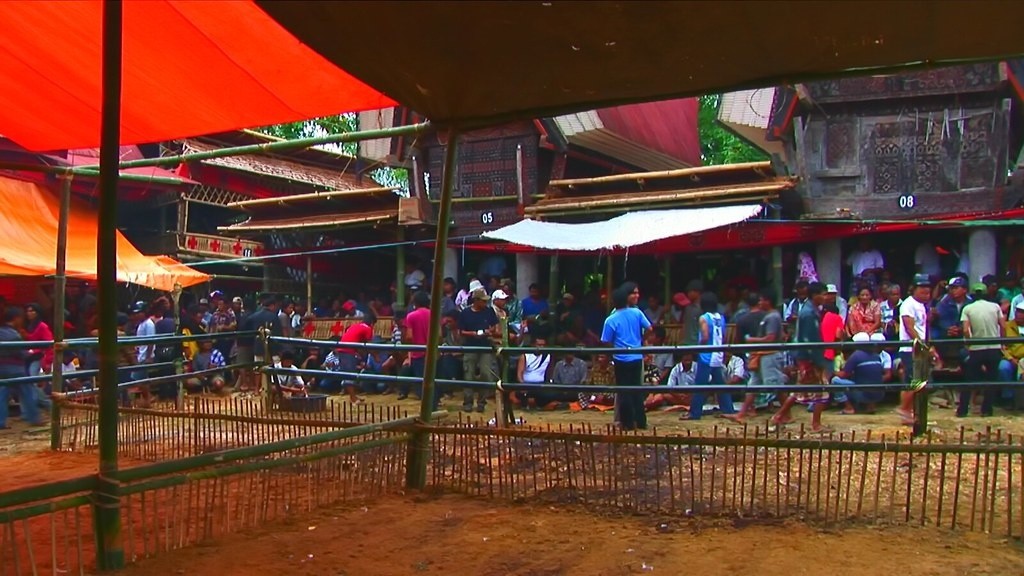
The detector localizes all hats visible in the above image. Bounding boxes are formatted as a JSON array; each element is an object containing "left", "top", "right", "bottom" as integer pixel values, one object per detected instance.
[
  {"left": 472, "top": 288, "right": 491, "bottom": 300},
  {"left": 232, "top": 297, "right": 242, "bottom": 303},
  {"left": 969, "top": 282, "right": 987, "bottom": 295},
  {"left": 469, "top": 280, "right": 484, "bottom": 293},
  {"left": 491, "top": 290, "right": 509, "bottom": 301},
  {"left": 672, "top": 292, "right": 691, "bottom": 306},
  {"left": 826, "top": 284, "right": 838, "bottom": 293},
  {"left": 200, "top": 298, "right": 208, "bottom": 303},
  {"left": 945, "top": 277, "right": 968, "bottom": 289}
]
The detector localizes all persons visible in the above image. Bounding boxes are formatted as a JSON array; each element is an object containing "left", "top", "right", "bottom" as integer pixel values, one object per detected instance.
[
  {"left": 601, "top": 281, "right": 653, "bottom": 429},
  {"left": 0, "top": 261, "right": 601, "bottom": 429},
  {"left": 642, "top": 233, "right": 1024, "bottom": 432}
]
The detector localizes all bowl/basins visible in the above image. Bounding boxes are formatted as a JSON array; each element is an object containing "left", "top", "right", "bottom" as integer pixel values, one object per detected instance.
[{"left": 284, "top": 394, "right": 329, "bottom": 413}]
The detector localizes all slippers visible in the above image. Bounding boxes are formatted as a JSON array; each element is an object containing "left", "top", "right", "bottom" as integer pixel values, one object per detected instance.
[
  {"left": 858, "top": 404, "right": 880, "bottom": 413},
  {"left": 833, "top": 408, "right": 857, "bottom": 414}
]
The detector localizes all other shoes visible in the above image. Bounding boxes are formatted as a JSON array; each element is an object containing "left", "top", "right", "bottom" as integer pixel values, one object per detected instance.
[
  {"left": 895, "top": 407, "right": 914, "bottom": 425},
  {"left": 397, "top": 394, "right": 408, "bottom": 400},
  {"left": 463, "top": 402, "right": 472, "bottom": 412},
  {"left": 478, "top": 405, "right": 484, "bottom": 412},
  {"left": 1001, "top": 397, "right": 1015, "bottom": 410},
  {"left": 679, "top": 415, "right": 700, "bottom": 420}
]
